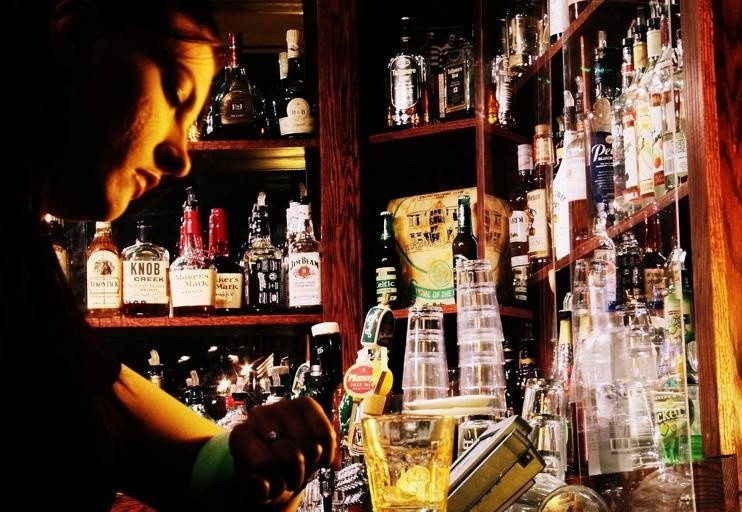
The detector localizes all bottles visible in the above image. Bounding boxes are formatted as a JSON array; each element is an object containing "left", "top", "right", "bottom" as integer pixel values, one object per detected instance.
[
  {"left": 41, "top": 182, "right": 328, "bottom": 318},
  {"left": 482, "top": 0, "right": 687, "bottom": 305},
  {"left": 144, "top": 348, "right": 324, "bottom": 437},
  {"left": 372, "top": 211, "right": 404, "bottom": 313},
  {"left": 452, "top": 194, "right": 480, "bottom": 305},
  {"left": 183, "top": 24, "right": 316, "bottom": 144},
  {"left": 379, "top": 15, "right": 475, "bottom": 130}
]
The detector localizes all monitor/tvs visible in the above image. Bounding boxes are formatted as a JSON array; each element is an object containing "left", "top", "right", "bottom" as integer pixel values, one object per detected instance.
[{"left": 448, "top": 414, "right": 547, "bottom": 512}]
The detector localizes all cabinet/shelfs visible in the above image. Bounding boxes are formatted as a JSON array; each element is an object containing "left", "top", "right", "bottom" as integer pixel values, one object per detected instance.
[
  {"left": 512, "top": 0, "right": 742, "bottom": 512},
  {"left": 79, "top": 3, "right": 363, "bottom": 436},
  {"left": 309, "top": 1, "right": 544, "bottom": 408}
]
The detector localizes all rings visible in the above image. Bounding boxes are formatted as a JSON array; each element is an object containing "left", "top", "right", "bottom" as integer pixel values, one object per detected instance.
[{"left": 265, "top": 424, "right": 284, "bottom": 445}]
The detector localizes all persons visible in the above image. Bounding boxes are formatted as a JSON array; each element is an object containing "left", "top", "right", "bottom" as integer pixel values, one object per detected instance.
[{"left": 0, "top": 2, "right": 337, "bottom": 511}]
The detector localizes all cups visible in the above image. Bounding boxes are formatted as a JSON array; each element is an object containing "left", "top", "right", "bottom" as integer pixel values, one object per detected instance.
[{"left": 340, "top": 259, "right": 698, "bottom": 511}]
